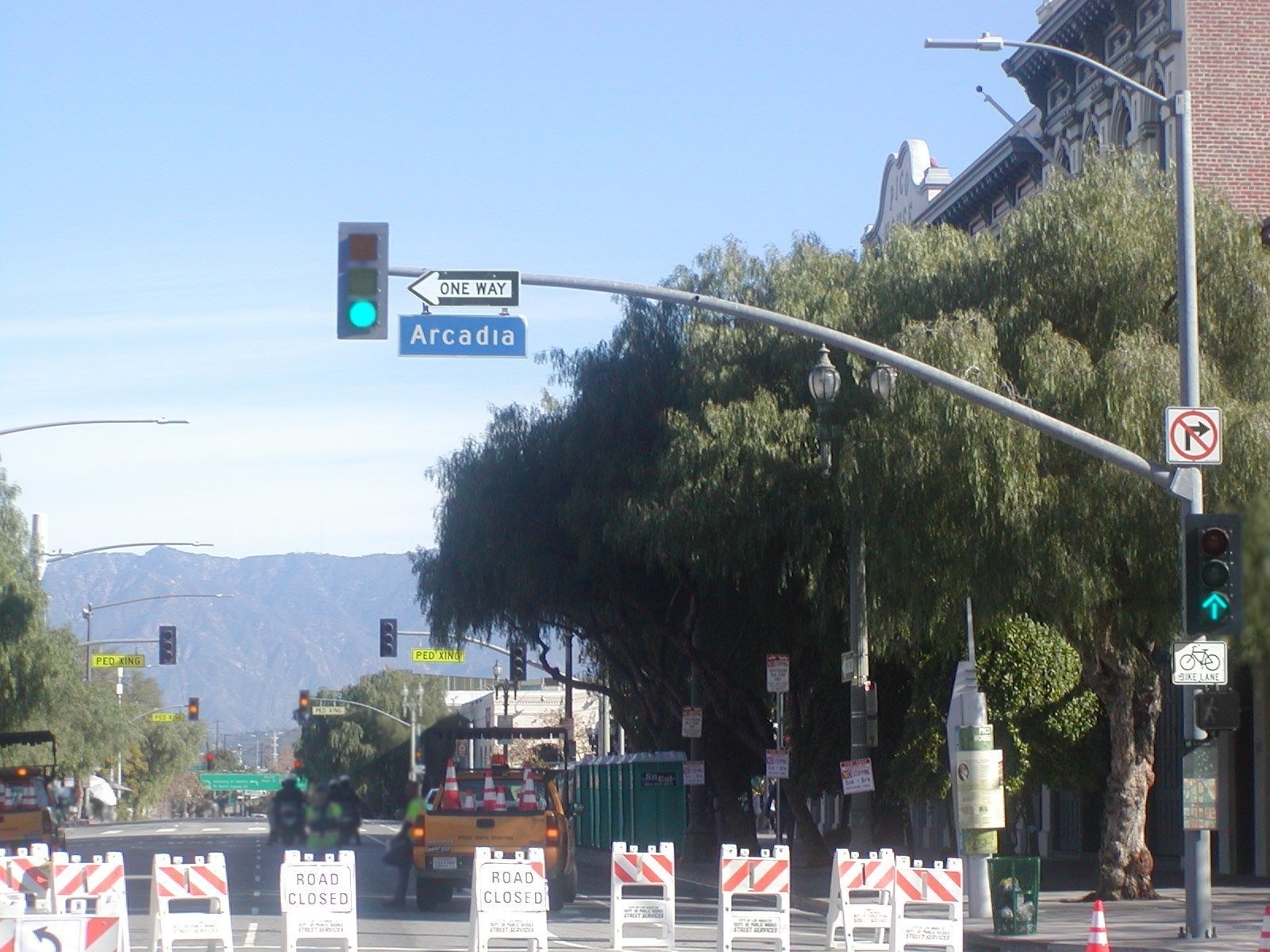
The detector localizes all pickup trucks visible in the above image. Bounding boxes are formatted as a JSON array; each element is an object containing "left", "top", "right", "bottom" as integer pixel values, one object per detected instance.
[
  {"left": 409, "top": 768, "right": 585, "bottom": 912},
  {"left": 0, "top": 731, "right": 65, "bottom": 856}
]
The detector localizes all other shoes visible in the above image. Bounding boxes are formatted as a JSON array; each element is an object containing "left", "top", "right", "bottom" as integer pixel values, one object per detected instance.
[{"left": 388, "top": 900, "right": 405, "bottom": 908}]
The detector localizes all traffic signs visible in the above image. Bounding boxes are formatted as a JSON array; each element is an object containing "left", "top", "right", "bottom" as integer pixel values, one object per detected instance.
[
  {"left": 312, "top": 706, "right": 345, "bottom": 715},
  {"left": 152, "top": 713, "right": 184, "bottom": 722},
  {"left": 198, "top": 774, "right": 309, "bottom": 792},
  {"left": 397, "top": 314, "right": 528, "bottom": 358},
  {"left": 406, "top": 269, "right": 521, "bottom": 308},
  {"left": 411, "top": 648, "right": 465, "bottom": 663},
  {"left": 1164, "top": 405, "right": 1223, "bottom": 466},
  {"left": 1171, "top": 641, "right": 1227, "bottom": 685},
  {"left": 91, "top": 655, "right": 145, "bottom": 667}
]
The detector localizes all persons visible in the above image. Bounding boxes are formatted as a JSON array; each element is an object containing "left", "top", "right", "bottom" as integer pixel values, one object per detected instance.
[
  {"left": 333, "top": 774, "right": 366, "bottom": 845},
  {"left": 329, "top": 779, "right": 340, "bottom": 800},
  {"left": 764, "top": 781, "right": 778, "bottom": 832},
  {"left": 266, "top": 775, "right": 309, "bottom": 844},
  {"left": 380, "top": 780, "right": 428, "bottom": 907},
  {"left": 303, "top": 784, "right": 342, "bottom": 862},
  {"left": 751, "top": 786, "right": 761, "bottom": 831}
]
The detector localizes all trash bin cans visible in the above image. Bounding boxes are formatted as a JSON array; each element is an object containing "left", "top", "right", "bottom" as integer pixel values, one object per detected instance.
[{"left": 987, "top": 855, "right": 1041, "bottom": 936}]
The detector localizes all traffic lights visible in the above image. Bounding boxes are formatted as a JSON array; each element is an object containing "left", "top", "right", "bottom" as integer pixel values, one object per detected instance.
[
  {"left": 293, "top": 750, "right": 305, "bottom": 776},
  {"left": 509, "top": 642, "right": 527, "bottom": 682},
  {"left": 299, "top": 690, "right": 310, "bottom": 723},
  {"left": 1184, "top": 514, "right": 1242, "bottom": 637},
  {"left": 206, "top": 751, "right": 214, "bottom": 771},
  {"left": 188, "top": 698, "right": 200, "bottom": 721},
  {"left": 159, "top": 625, "right": 177, "bottom": 665},
  {"left": 338, "top": 222, "right": 389, "bottom": 341},
  {"left": 380, "top": 618, "right": 398, "bottom": 658}
]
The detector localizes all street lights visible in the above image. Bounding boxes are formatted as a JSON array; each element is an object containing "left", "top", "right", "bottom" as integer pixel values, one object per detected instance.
[
  {"left": 493, "top": 660, "right": 519, "bottom": 767},
  {"left": 81, "top": 594, "right": 234, "bottom": 681},
  {"left": 923, "top": 32, "right": 1220, "bottom": 943}
]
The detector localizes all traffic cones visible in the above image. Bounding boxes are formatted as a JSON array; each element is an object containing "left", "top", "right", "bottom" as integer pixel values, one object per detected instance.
[
  {"left": 442, "top": 759, "right": 461, "bottom": 810},
  {"left": 1085, "top": 900, "right": 1111, "bottom": 952},
  {"left": 493, "top": 786, "right": 507, "bottom": 812},
  {"left": 464, "top": 789, "right": 474, "bottom": 810},
  {"left": 483, "top": 770, "right": 497, "bottom": 811},
  {"left": 518, "top": 763, "right": 539, "bottom": 811},
  {"left": 1256, "top": 906, "right": 1270, "bottom": 952}
]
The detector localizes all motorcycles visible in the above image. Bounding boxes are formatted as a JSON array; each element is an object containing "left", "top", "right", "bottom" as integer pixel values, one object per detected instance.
[{"left": 269, "top": 796, "right": 354, "bottom": 845}]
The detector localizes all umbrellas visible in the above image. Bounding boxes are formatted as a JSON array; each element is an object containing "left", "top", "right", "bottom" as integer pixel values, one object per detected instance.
[
  {"left": 108, "top": 781, "right": 132, "bottom": 791},
  {"left": 89, "top": 773, "right": 117, "bottom": 807}
]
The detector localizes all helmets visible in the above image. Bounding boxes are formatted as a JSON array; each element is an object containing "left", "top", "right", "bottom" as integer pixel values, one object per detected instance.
[
  {"left": 329, "top": 779, "right": 338, "bottom": 786},
  {"left": 54, "top": 787, "right": 71, "bottom": 804},
  {"left": 489, "top": 755, "right": 508, "bottom": 767},
  {"left": 284, "top": 774, "right": 296, "bottom": 782},
  {"left": 340, "top": 775, "right": 351, "bottom": 781}
]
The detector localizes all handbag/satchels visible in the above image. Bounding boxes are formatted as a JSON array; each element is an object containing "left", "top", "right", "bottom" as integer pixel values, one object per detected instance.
[{"left": 380, "top": 834, "right": 414, "bottom": 868}]
